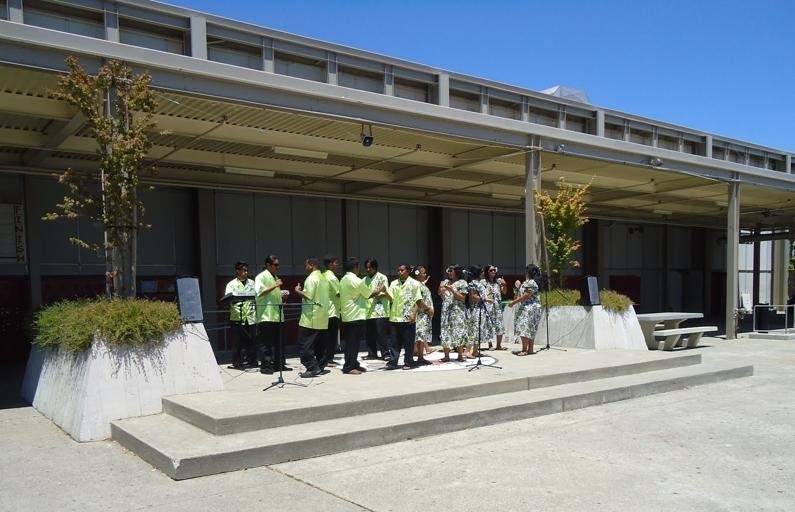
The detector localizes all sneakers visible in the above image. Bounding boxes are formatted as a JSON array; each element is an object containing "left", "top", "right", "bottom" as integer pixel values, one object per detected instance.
[
  {"left": 518, "top": 350, "right": 533, "bottom": 355},
  {"left": 489, "top": 346, "right": 507, "bottom": 351},
  {"left": 438, "top": 346, "right": 484, "bottom": 362}
]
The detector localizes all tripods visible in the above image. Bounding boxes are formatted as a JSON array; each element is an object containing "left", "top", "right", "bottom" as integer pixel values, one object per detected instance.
[
  {"left": 460, "top": 288, "right": 501, "bottom": 373},
  {"left": 263, "top": 301, "right": 316, "bottom": 393},
  {"left": 531, "top": 278, "right": 567, "bottom": 353},
  {"left": 228, "top": 305, "right": 261, "bottom": 372}
]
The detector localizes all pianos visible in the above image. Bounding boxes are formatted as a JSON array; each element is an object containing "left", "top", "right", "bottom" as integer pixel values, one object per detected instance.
[{"left": 219, "top": 290, "right": 290, "bottom": 302}]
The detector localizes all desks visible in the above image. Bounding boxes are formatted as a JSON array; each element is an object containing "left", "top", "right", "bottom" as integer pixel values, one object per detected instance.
[{"left": 637, "top": 313, "right": 703, "bottom": 349}]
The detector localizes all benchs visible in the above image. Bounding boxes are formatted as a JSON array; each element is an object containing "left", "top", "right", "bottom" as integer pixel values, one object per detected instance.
[
  {"left": 653, "top": 325, "right": 718, "bottom": 350},
  {"left": 655, "top": 325, "right": 664, "bottom": 329}
]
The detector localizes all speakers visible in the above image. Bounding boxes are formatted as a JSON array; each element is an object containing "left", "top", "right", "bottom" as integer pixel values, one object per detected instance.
[
  {"left": 579, "top": 275, "right": 601, "bottom": 305},
  {"left": 175, "top": 274, "right": 206, "bottom": 323}
]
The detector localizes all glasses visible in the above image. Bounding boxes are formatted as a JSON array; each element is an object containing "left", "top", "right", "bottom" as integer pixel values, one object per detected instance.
[{"left": 489, "top": 268, "right": 496, "bottom": 272}]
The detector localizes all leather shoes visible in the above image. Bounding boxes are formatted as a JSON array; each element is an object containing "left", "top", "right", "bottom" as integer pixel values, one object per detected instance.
[
  {"left": 344, "top": 367, "right": 365, "bottom": 374},
  {"left": 260, "top": 364, "right": 292, "bottom": 374},
  {"left": 233, "top": 361, "right": 258, "bottom": 370},
  {"left": 300, "top": 359, "right": 336, "bottom": 378},
  {"left": 384, "top": 348, "right": 439, "bottom": 370},
  {"left": 362, "top": 354, "right": 377, "bottom": 359}
]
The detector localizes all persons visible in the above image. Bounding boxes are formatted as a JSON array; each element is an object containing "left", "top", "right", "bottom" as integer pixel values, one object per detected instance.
[
  {"left": 225, "top": 259, "right": 258, "bottom": 369},
  {"left": 294, "top": 256, "right": 543, "bottom": 378},
  {"left": 254, "top": 254, "right": 289, "bottom": 374}
]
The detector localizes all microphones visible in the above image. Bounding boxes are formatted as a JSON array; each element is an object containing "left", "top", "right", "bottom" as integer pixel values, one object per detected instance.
[{"left": 461, "top": 268, "right": 474, "bottom": 278}]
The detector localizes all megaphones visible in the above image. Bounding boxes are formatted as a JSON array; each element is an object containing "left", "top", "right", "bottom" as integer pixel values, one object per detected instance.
[{"left": 359, "top": 132, "right": 374, "bottom": 146}]
